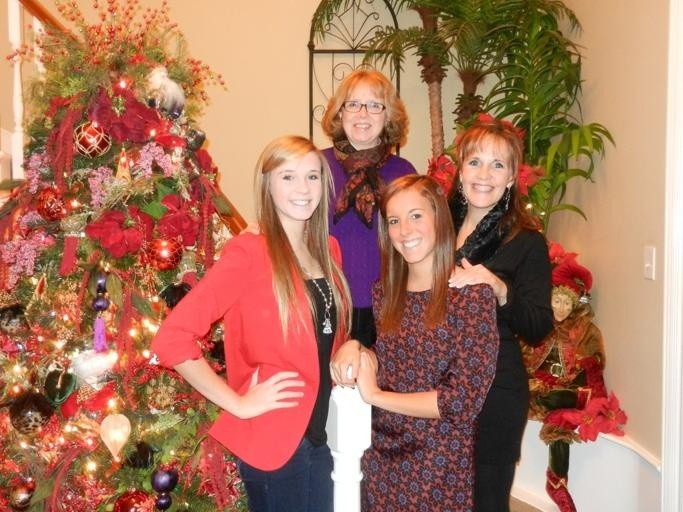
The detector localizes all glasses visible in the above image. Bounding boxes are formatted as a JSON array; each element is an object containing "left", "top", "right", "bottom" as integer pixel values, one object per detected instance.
[{"left": 343, "top": 101, "right": 386, "bottom": 114}]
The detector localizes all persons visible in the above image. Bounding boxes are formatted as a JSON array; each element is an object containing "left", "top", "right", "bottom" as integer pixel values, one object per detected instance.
[
  {"left": 150, "top": 136, "right": 356, "bottom": 512},
  {"left": 329, "top": 173, "right": 501, "bottom": 512},
  {"left": 520, "top": 247, "right": 610, "bottom": 511},
  {"left": 318, "top": 70, "right": 418, "bottom": 349},
  {"left": 438, "top": 115, "right": 552, "bottom": 510}
]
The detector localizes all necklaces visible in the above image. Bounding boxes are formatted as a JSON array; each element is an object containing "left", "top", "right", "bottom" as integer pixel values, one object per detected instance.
[{"left": 296, "top": 255, "right": 334, "bottom": 335}]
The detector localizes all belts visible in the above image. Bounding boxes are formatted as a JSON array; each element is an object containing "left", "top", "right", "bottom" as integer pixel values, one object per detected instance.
[{"left": 543, "top": 361, "right": 563, "bottom": 378}]
